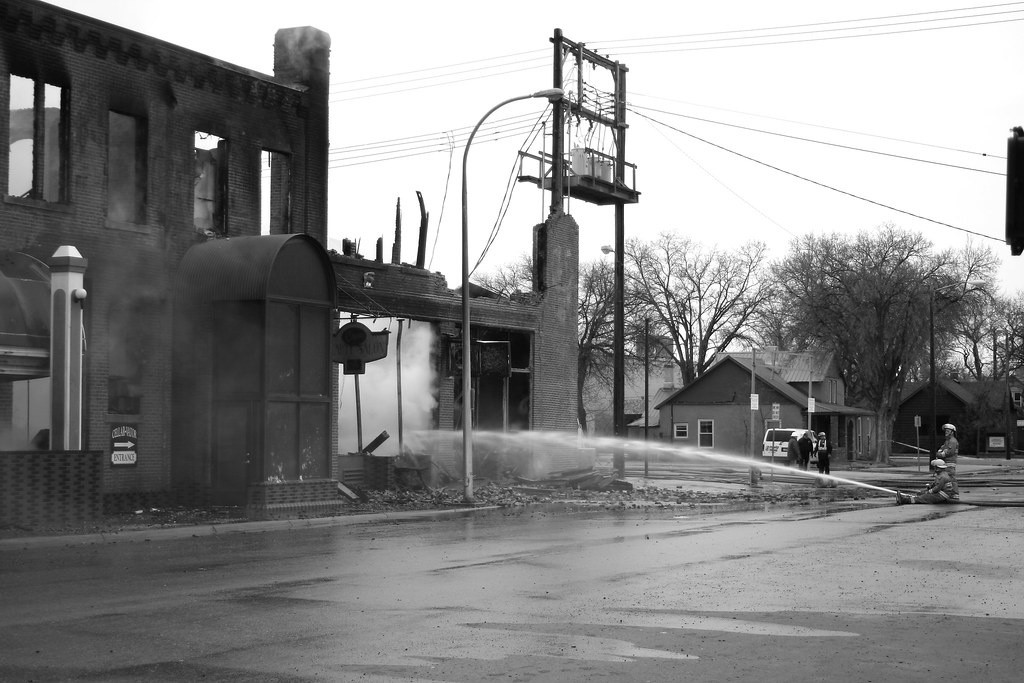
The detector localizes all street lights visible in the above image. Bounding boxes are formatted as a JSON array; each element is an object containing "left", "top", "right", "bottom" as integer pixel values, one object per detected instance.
[
  {"left": 928, "top": 280, "right": 986, "bottom": 466},
  {"left": 461, "top": 89, "right": 565, "bottom": 500}
]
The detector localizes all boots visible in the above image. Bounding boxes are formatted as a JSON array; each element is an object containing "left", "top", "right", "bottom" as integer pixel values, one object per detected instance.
[{"left": 897, "top": 491, "right": 911, "bottom": 505}]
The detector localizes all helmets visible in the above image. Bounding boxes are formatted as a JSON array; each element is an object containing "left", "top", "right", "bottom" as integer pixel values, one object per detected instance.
[
  {"left": 818, "top": 432, "right": 825, "bottom": 437},
  {"left": 944, "top": 424, "right": 956, "bottom": 431},
  {"left": 790, "top": 432, "right": 799, "bottom": 437},
  {"left": 931, "top": 458, "right": 947, "bottom": 469}
]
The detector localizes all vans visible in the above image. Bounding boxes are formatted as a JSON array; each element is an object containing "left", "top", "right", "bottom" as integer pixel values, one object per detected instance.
[{"left": 761, "top": 427, "right": 819, "bottom": 464}]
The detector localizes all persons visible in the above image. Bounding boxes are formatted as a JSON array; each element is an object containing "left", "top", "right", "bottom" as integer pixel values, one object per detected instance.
[
  {"left": 788, "top": 432, "right": 802, "bottom": 468},
  {"left": 936, "top": 424, "right": 959, "bottom": 499},
  {"left": 814, "top": 432, "right": 832, "bottom": 475},
  {"left": 895, "top": 459, "right": 952, "bottom": 506},
  {"left": 798, "top": 432, "right": 814, "bottom": 471}
]
